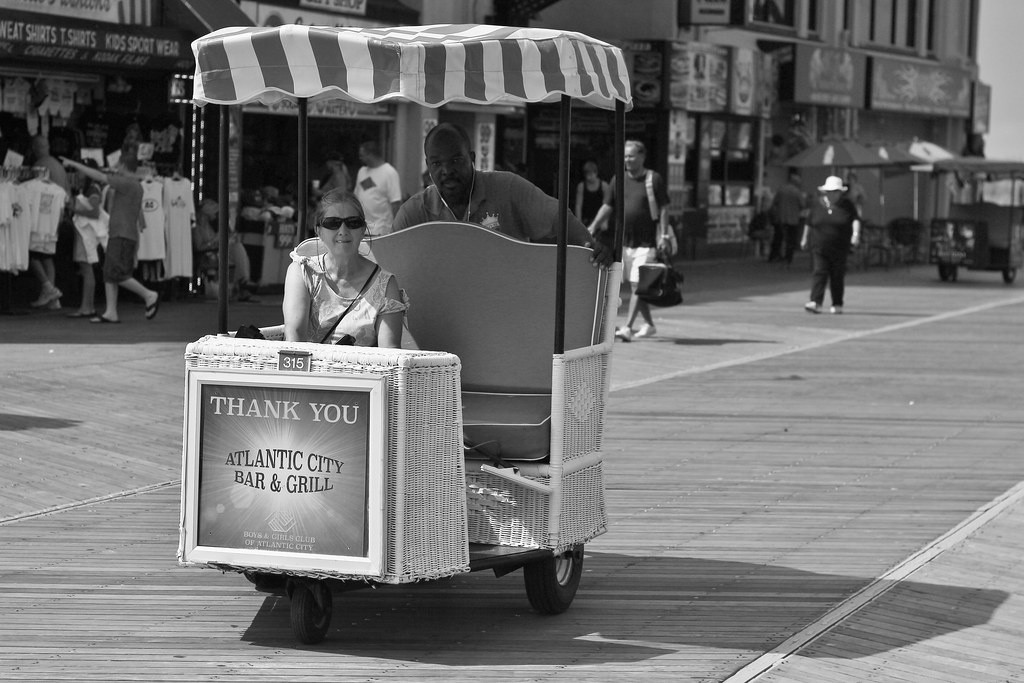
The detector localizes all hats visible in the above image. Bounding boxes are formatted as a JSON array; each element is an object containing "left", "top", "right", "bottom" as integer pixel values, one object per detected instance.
[{"left": 817, "top": 176, "right": 848, "bottom": 193}]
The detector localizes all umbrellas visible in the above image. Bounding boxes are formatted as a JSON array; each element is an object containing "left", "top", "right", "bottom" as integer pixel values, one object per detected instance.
[
  {"left": 865, "top": 139, "right": 929, "bottom": 263},
  {"left": 784, "top": 135, "right": 894, "bottom": 176},
  {"left": 885, "top": 136, "right": 959, "bottom": 259}
]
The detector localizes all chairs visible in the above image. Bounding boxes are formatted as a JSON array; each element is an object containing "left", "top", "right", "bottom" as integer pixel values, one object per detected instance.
[{"left": 863, "top": 218, "right": 925, "bottom": 269}]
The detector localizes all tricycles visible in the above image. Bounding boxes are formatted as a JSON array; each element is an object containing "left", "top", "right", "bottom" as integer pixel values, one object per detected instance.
[
  {"left": 928, "top": 157, "right": 1023, "bottom": 286},
  {"left": 177, "top": 22, "right": 639, "bottom": 646}
]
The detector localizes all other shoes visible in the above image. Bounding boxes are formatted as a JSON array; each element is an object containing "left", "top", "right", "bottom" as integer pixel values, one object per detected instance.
[
  {"left": 635, "top": 323, "right": 657, "bottom": 338},
  {"left": 805, "top": 300, "right": 823, "bottom": 314},
  {"left": 782, "top": 256, "right": 792, "bottom": 265},
  {"left": 830, "top": 306, "right": 841, "bottom": 314},
  {"left": 768, "top": 254, "right": 780, "bottom": 263},
  {"left": 240, "top": 279, "right": 258, "bottom": 288},
  {"left": 614, "top": 325, "right": 631, "bottom": 342},
  {"left": 42, "top": 299, "right": 62, "bottom": 310},
  {"left": 238, "top": 293, "right": 262, "bottom": 303},
  {"left": 31, "top": 287, "right": 63, "bottom": 307}
]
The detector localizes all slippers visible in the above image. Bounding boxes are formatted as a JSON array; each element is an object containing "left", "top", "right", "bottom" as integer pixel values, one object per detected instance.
[
  {"left": 90, "top": 315, "right": 121, "bottom": 324},
  {"left": 66, "top": 308, "right": 97, "bottom": 318},
  {"left": 145, "top": 292, "right": 160, "bottom": 319}
]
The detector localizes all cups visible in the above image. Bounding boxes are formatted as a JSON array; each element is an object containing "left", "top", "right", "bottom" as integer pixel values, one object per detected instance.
[{"left": 312, "top": 180, "right": 320, "bottom": 189}]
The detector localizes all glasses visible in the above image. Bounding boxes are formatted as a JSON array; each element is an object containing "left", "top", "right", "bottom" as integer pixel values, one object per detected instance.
[{"left": 317, "top": 216, "right": 365, "bottom": 230}]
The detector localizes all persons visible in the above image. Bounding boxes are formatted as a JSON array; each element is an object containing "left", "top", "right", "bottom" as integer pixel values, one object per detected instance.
[
  {"left": 58, "top": 153, "right": 165, "bottom": 324},
  {"left": 64, "top": 157, "right": 102, "bottom": 318},
  {"left": 194, "top": 199, "right": 252, "bottom": 302},
  {"left": 282, "top": 186, "right": 403, "bottom": 349},
  {"left": 576, "top": 162, "right": 608, "bottom": 237},
  {"left": 391, "top": 122, "right": 614, "bottom": 272},
  {"left": 28, "top": 135, "right": 72, "bottom": 309},
  {"left": 312, "top": 151, "right": 352, "bottom": 200},
  {"left": 801, "top": 175, "right": 864, "bottom": 314},
  {"left": 354, "top": 140, "right": 402, "bottom": 237},
  {"left": 587, "top": 141, "right": 672, "bottom": 342},
  {"left": 749, "top": 172, "right": 866, "bottom": 265}
]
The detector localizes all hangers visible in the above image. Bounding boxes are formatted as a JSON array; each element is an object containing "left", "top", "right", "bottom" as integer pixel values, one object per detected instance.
[
  {"left": 0, "top": 166, "right": 61, "bottom": 192},
  {"left": 6, "top": 68, "right": 77, "bottom": 90},
  {"left": 135, "top": 162, "right": 190, "bottom": 185}
]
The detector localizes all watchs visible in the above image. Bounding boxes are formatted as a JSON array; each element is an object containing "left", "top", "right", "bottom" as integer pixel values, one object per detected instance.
[{"left": 660, "top": 235, "right": 671, "bottom": 241}]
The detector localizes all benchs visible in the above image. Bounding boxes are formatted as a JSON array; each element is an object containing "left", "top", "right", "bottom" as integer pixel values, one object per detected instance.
[{"left": 227, "top": 219, "right": 609, "bottom": 461}]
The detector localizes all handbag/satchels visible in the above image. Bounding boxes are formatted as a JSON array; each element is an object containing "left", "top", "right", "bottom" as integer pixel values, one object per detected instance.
[
  {"left": 635, "top": 250, "right": 684, "bottom": 308},
  {"left": 655, "top": 223, "right": 678, "bottom": 257}
]
